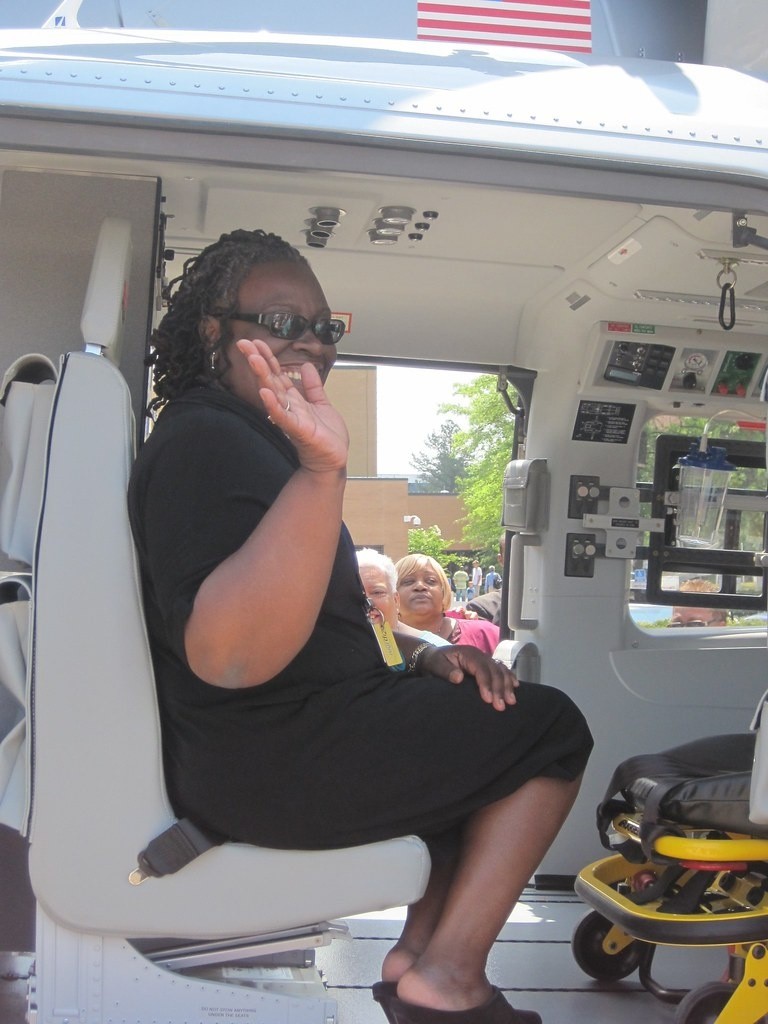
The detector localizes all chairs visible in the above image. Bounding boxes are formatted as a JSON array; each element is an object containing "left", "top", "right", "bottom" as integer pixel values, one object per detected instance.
[{"left": 0, "top": 213, "right": 432, "bottom": 1024}]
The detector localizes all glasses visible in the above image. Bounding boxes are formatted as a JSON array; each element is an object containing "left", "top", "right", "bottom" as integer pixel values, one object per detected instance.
[
  {"left": 211, "top": 311, "right": 345, "bottom": 345},
  {"left": 666, "top": 615, "right": 721, "bottom": 629}
]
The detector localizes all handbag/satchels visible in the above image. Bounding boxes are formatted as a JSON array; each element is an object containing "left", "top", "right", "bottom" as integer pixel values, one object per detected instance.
[{"left": 494, "top": 573, "right": 503, "bottom": 590}]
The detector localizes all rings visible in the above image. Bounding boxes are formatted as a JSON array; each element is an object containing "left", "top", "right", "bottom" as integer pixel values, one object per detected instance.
[{"left": 267, "top": 402, "right": 291, "bottom": 424}]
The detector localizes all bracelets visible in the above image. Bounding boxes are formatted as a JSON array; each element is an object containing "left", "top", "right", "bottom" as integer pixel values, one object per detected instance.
[{"left": 408, "top": 643, "right": 436, "bottom": 673}]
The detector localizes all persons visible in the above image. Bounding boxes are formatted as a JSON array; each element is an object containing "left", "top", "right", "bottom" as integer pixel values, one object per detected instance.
[
  {"left": 128, "top": 227, "right": 597, "bottom": 1024},
  {"left": 671, "top": 579, "right": 728, "bottom": 629},
  {"left": 354, "top": 532, "right": 515, "bottom": 659}
]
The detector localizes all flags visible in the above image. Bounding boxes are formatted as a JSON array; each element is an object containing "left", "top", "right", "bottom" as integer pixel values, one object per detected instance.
[{"left": 417, "top": 0, "right": 592, "bottom": 53}]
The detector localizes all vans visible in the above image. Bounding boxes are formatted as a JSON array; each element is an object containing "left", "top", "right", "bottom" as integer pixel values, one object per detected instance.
[{"left": 0, "top": 5, "right": 766, "bottom": 1024}]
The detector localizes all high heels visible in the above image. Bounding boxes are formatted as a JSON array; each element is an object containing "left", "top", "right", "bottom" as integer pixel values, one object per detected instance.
[{"left": 372, "top": 981, "right": 542, "bottom": 1024}]
